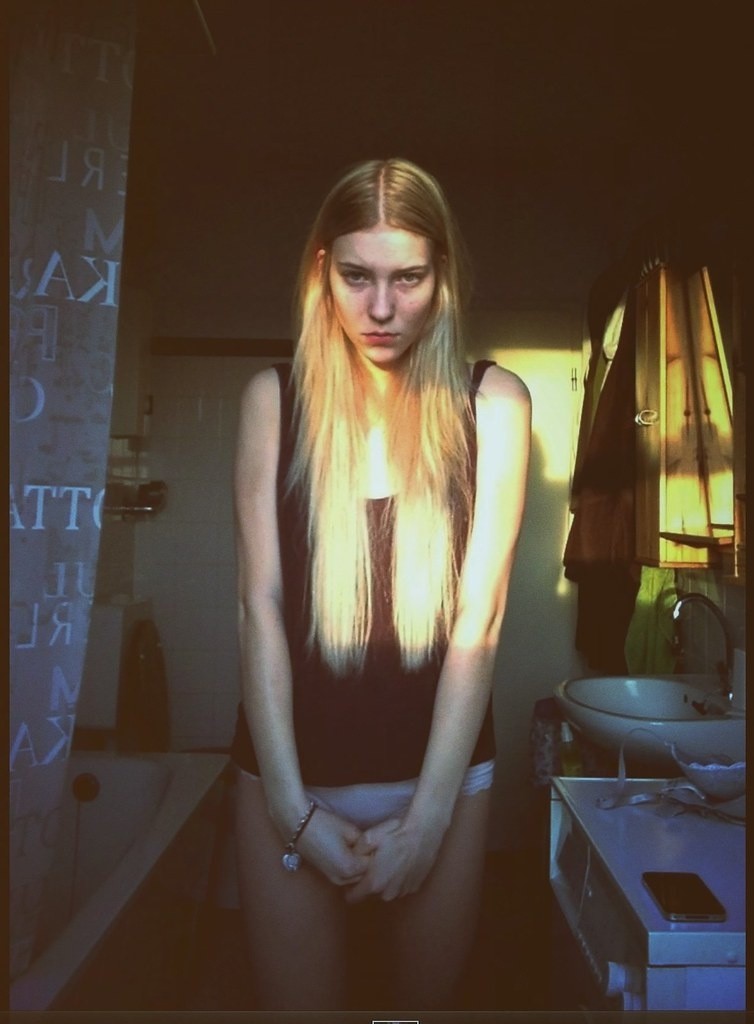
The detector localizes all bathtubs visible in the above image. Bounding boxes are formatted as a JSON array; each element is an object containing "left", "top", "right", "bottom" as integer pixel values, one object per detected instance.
[{"left": 9, "top": 750, "right": 230, "bottom": 1019}]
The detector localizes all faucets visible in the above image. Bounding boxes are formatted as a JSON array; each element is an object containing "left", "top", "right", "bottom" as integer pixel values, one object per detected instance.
[{"left": 669, "top": 593, "right": 734, "bottom": 705}]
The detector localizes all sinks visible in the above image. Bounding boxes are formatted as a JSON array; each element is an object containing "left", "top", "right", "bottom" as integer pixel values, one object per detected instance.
[{"left": 556, "top": 676, "right": 745, "bottom": 761}]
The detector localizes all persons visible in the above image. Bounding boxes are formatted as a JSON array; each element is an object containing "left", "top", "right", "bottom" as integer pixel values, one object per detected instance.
[{"left": 233, "top": 160, "right": 532, "bottom": 1011}]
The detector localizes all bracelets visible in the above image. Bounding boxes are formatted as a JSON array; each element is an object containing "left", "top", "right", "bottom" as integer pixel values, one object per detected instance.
[{"left": 282, "top": 802, "right": 317, "bottom": 872}]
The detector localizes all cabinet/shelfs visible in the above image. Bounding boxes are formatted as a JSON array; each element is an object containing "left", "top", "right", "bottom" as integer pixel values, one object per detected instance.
[{"left": 549, "top": 775, "right": 747, "bottom": 1012}]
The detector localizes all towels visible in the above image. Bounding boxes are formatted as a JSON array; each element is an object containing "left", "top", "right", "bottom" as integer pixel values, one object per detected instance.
[
  {"left": 115, "top": 620, "right": 171, "bottom": 751},
  {"left": 561, "top": 280, "right": 674, "bottom": 674}
]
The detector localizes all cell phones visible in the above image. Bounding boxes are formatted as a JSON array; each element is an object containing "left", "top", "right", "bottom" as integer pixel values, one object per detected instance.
[{"left": 641, "top": 871, "right": 727, "bottom": 923}]
